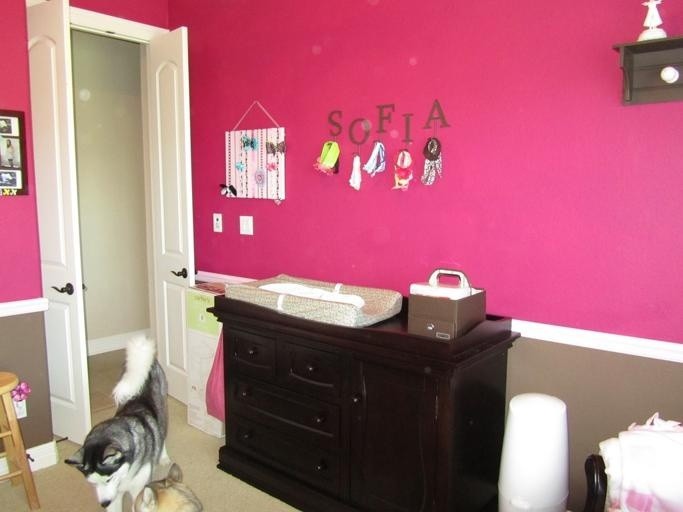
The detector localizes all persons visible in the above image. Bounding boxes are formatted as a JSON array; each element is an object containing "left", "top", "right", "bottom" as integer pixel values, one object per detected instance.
[{"left": 6, "top": 140, "right": 14, "bottom": 167}]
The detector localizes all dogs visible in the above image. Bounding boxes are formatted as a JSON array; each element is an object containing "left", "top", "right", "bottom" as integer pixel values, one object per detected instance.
[{"left": 64, "top": 334, "right": 202, "bottom": 512}]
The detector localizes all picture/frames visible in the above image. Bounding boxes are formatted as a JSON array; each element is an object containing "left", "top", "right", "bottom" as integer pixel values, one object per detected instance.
[{"left": 0, "top": 110, "right": 29, "bottom": 196}]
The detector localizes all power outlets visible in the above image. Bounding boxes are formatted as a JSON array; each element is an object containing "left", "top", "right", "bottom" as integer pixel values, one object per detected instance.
[{"left": 213, "top": 213, "right": 222, "bottom": 233}]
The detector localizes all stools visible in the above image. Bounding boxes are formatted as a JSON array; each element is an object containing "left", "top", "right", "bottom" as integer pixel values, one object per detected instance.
[{"left": 0, "top": 370, "right": 41, "bottom": 511}]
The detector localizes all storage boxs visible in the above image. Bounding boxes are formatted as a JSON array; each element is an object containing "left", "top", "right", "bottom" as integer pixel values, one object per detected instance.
[{"left": 407, "top": 269, "right": 486, "bottom": 339}]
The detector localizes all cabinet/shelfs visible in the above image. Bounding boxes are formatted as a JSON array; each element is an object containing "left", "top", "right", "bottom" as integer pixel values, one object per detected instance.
[
  {"left": 206, "top": 296, "right": 521, "bottom": 512},
  {"left": 186, "top": 282, "right": 235, "bottom": 439}
]
